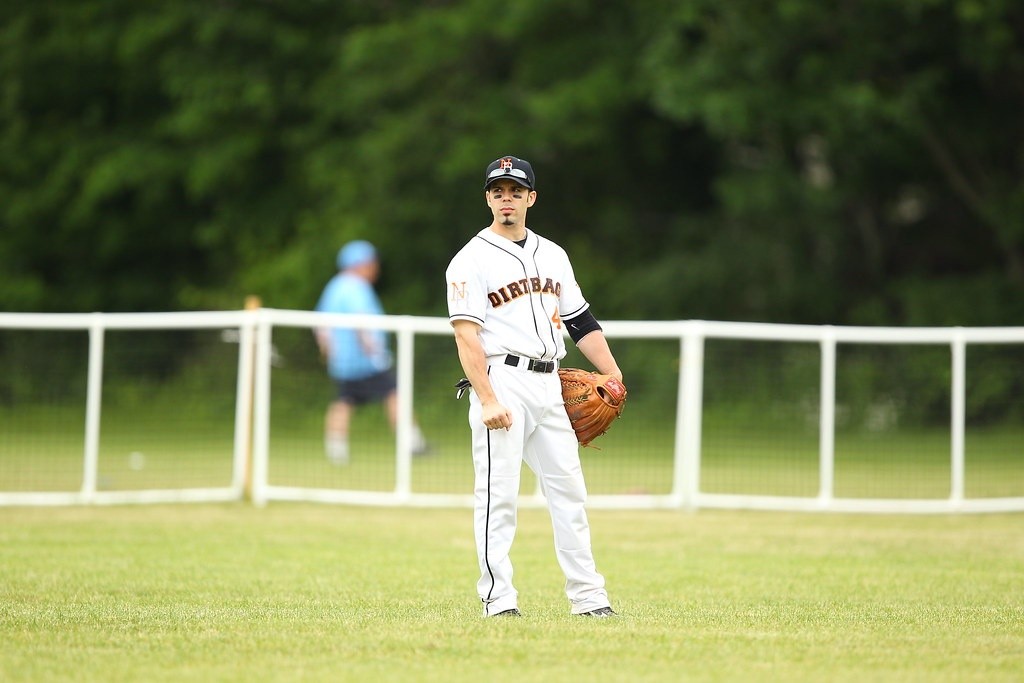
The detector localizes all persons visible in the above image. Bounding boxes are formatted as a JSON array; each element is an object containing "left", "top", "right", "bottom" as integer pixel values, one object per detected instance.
[
  {"left": 311, "top": 240, "right": 427, "bottom": 467},
  {"left": 444, "top": 155, "right": 623, "bottom": 618}
]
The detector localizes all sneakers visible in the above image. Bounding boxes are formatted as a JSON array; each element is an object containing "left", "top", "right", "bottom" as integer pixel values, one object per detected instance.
[
  {"left": 494, "top": 609, "right": 521, "bottom": 616},
  {"left": 579, "top": 607, "right": 617, "bottom": 617}
]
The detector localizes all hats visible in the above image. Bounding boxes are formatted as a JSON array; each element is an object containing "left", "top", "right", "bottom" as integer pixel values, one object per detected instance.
[
  {"left": 484, "top": 156, "right": 535, "bottom": 191},
  {"left": 337, "top": 240, "right": 376, "bottom": 269}
]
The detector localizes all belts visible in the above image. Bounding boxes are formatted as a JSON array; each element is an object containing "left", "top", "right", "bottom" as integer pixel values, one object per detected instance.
[{"left": 505, "top": 354, "right": 558, "bottom": 373}]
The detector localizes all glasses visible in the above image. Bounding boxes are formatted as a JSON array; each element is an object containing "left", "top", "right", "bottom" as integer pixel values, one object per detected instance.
[{"left": 485, "top": 168, "right": 530, "bottom": 187}]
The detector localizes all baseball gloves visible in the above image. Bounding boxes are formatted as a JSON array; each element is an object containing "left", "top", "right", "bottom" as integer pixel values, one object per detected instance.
[{"left": 557, "top": 365, "right": 628, "bottom": 448}]
